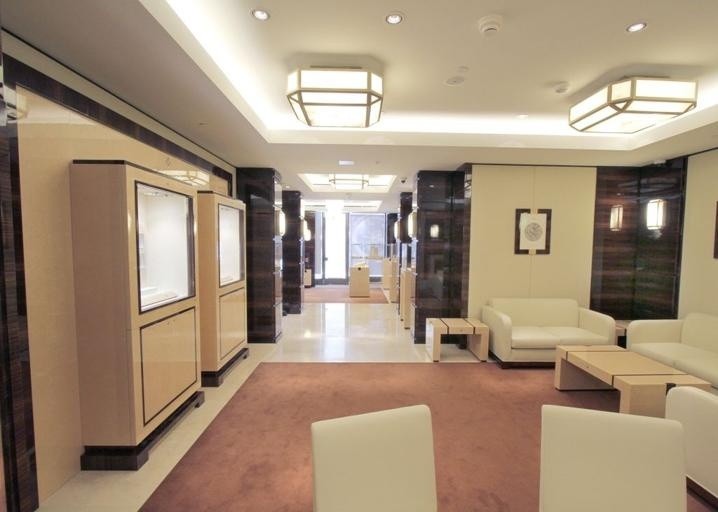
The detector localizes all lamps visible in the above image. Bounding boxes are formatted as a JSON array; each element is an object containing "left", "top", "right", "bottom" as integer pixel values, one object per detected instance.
[
  {"left": 568, "top": 75, "right": 698, "bottom": 135},
  {"left": 328, "top": 174, "right": 369, "bottom": 190},
  {"left": 646, "top": 198, "right": 664, "bottom": 230},
  {"left": 609, "top": 205, "right": 623, "bottom": 232},
  {"left": 284, "top": 65, "right": 384, "bottom": 129},
  {"left": 157, "top": 169, "right": 209, "bottom": 186}
]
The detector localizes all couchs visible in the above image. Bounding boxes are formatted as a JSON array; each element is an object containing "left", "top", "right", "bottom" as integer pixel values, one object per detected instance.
[
  {"left": 626, "top": 312, "right": 718, "bottom": 396},
  {"left": 665, "top": 386, "right": 718, "bottom": 510},
  {"left": 482, "top": 297, "right": 616, "bottom": 369}
]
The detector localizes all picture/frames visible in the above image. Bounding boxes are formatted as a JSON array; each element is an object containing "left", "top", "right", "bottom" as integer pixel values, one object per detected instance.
[{"left": 514, "top": 208, "right": 552, "bottom": 254}]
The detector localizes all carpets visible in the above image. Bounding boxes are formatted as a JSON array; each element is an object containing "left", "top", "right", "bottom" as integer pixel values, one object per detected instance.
[
  {"left": 303, "top": 288, "right": 388, "bottom": 304},
  {"left": 137, "top": 362, "right": 718, "bottom": 512}
]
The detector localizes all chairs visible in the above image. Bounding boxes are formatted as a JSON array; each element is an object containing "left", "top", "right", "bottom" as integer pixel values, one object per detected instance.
[
  {"left": 539, "top": 404, "right": 687, "bottom": 512},
  {"left": 310, "top": 404, "right": 438, "bottom": 512}
]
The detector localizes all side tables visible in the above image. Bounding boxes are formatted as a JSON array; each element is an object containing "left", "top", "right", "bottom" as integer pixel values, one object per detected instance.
[
  {"left": 425, "top": 318, "right": 489, "bottom": 362},
  {"left": 615, "top": 320, "right": 631, "bottom": 345}
]
